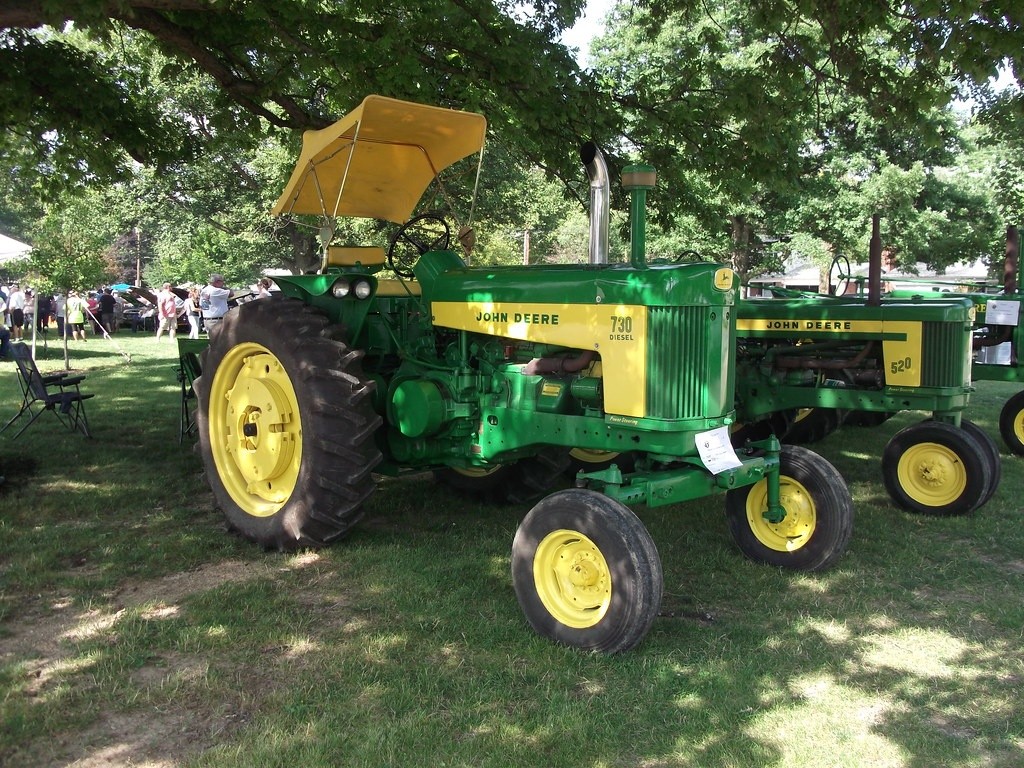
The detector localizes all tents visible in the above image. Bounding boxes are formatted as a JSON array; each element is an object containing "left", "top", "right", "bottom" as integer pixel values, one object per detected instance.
[{"left": 0, "top": 233, "right": 37, "bottom": 363}]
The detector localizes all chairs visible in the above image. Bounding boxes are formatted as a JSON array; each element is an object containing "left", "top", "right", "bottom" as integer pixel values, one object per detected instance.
[
  {"left": 0, "top": 340, "right": 96, "bottom": 440},
  {"left": 169, "top": 338, "right": 212, "bottom": 443},
  {"left": 323, "top": 245, "right": 423, "bottom": 315}
]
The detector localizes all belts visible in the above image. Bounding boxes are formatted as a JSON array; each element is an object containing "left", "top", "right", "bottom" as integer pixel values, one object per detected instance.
[{"left": 204, "top": 317, "right": 223, "bottom": 320}]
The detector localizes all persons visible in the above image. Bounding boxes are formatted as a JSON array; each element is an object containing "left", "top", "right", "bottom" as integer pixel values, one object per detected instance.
[{"left": 0, "top": 273, "right": 273, "bottom": 341}]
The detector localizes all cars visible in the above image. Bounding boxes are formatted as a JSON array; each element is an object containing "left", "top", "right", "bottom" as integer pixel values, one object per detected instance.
[{"left": 113, "top": 286, "right": 190, "bottom": 330}]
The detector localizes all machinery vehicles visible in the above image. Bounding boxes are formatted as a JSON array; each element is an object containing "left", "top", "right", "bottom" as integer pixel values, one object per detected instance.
[
  {"left": 191, "top": 95, "right": 855, "bottom": 658},
  {"left": 736, "top": 256, "right": 1024, "bottom": 516}
]
